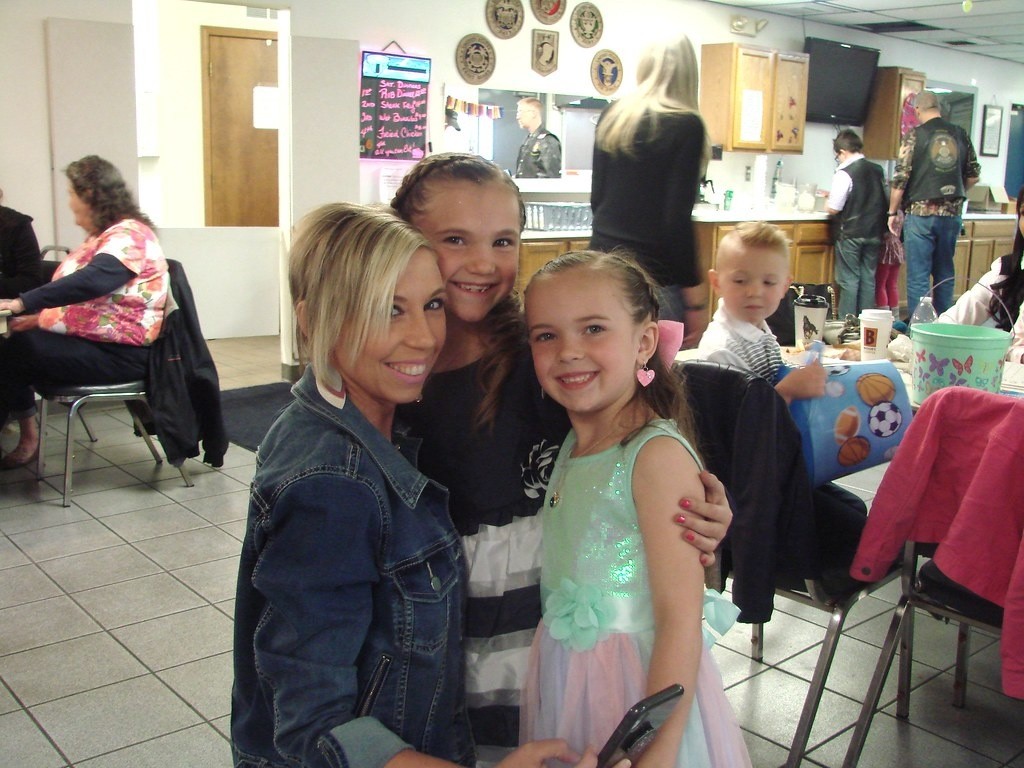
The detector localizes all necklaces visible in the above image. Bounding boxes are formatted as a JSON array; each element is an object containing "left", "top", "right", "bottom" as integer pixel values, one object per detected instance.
[{"left": 549, "top": 427, "right": 621, "bottom": 507}]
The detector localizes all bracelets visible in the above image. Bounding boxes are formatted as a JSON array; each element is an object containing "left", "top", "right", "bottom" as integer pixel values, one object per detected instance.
[{"left": 888, "top": 212, "right": 897, "bottom": 216}]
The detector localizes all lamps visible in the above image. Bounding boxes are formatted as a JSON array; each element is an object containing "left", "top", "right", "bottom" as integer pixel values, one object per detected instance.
[{"left": 730, "top": 15, "right": 769, "bottom": 37}]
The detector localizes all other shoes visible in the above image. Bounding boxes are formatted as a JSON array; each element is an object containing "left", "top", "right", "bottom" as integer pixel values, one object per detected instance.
[{"left": 0, "top": 439, "right": 39, "bottom": 469}]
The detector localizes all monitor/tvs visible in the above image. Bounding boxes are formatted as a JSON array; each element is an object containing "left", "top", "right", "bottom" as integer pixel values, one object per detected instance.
[{"left": 804, "top": 36, "right": 881, "bottom": 126}]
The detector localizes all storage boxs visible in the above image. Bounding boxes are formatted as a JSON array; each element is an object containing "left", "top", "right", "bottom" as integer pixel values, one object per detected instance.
[
  {"left": 1002, "top": 198, "right": 1017, "bottom": 215},
  {"left": 966, "top": 185, "right": 1008, "bottom": 216}
]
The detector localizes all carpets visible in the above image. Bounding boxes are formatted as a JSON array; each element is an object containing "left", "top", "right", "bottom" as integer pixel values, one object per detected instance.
[{"left": 220, "top": 382, "right": 294, "bottom": 453}]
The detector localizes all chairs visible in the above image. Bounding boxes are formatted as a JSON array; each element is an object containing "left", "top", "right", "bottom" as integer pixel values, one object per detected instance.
[
  {"left": 672, "top": 362, "right": 913, "bottom": 768},
  {"left": 844, "top": 386, "right": 1024, "bottom": 768},
  {"left": 36, "top": 246, "right": 195, "bottom": 507}
]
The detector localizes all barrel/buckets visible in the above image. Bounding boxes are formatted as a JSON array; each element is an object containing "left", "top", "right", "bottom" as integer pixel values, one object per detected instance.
[{"left": 908, "top": 275, "right": 1015, "bottom": 412}]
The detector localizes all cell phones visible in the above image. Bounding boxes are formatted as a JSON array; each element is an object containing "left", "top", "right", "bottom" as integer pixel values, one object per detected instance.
[{"left": 595, "top": 683, "right": 685, "bottom": 768}]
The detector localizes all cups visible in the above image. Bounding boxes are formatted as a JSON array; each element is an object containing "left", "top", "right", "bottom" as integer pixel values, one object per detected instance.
[
  {"left": 750, "top": 153, "right": 818, "bottom": 214},
  {"left": 792, "top": 294, "right": 830, "bottom": 350},
  {"left": 857, "top": 309, "right": 895, "bottom": 364}
]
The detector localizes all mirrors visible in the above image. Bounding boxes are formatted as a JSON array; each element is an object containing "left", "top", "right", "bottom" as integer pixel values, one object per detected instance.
[
  {"left": 926, "top": 81, "right": 979, "bottom": 143},
  {"left": 477, "top": 88, "right": 610, "bottom": 178}
]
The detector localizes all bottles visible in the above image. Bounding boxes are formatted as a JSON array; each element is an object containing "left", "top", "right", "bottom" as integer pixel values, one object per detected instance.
[
  {"left": 909, "top": 296, "right": 939, "bottom": 334},
  {"left": 722, "top": 190, "right": 734, "bottom": 211}
]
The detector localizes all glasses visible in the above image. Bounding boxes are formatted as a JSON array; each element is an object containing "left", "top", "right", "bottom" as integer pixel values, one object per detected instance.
[{"left": 835, "top": 152, "right": 841, "bottom": 162}]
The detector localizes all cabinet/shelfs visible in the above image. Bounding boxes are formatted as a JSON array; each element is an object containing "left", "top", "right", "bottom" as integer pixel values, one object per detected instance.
[{"left": 517, "top": 41, "right": 1024, "bottom": 328}]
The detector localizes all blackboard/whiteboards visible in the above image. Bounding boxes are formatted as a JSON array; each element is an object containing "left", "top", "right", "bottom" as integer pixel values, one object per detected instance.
[{"left": 359, "top": 51, "right": 430, "bottom": 161}]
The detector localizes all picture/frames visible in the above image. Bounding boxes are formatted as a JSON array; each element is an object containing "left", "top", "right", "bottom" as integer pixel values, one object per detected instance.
[{"left": 980, "top": 104, "right": 1004, "bottom": 157}]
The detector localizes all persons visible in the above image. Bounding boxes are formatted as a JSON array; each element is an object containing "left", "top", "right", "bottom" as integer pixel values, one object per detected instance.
[
  {"left": 589, "top": 31, "right": 706, "bottom": 348},
  {"left": 230, "top": 199, "right": 630, "bottom": 768},
  {"left": 697, "top": 218, "right": 868, "bottom": 516},
  {"left": 391, "top": 152, "right": 733, "bottom": 768},
  {"left": 515, "top": 97, "right": 562, "bottom": 179},
  {"left": 825, "top": 128, "right": 889, "bottom": 319},
  {"left": 887, "top": 89, "right": 982, "bottom": 318},
  {"left": 0, "top": 154, "right": 170, "bottom": 469},
  {"left": 467, "top": 247, "right": 752, "bottom": 768},
  {"left": 929, "top": 188, "right": 1024, "bottom": 363},
  {"left": 874, "top": 168, "right": 905, "bottom": 319}
]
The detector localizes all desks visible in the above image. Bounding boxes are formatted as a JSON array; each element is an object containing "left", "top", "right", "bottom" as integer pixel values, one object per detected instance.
[{"left": 674, "top": 345, "right": 1024, "bottom": 408}]
